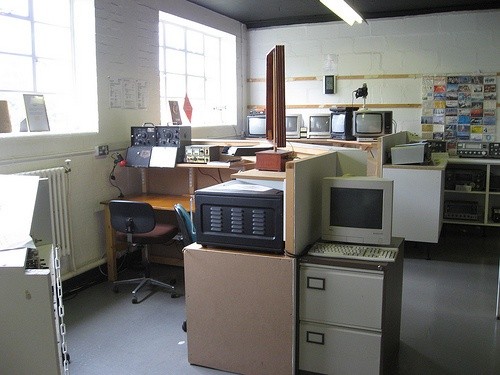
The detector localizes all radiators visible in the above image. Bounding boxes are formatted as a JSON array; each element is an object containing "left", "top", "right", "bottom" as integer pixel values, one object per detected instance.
[{"left": 14, "top": 158, "right": 78, "bottom": 273}]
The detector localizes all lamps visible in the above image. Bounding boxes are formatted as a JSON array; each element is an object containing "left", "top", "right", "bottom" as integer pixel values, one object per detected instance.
[{"left": 319, "top": 0, "right": 369, "bottom": 26}]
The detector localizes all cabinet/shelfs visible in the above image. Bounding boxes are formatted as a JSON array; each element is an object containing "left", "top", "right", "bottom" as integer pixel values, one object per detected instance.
[
  {"left": 443, "top": 150, "right": 500, "bottom": 237},
  {"left": 296, "top": 235, "right": 407, "bottom": 375},
  {"left": 382, "top": 151, "right": 451, "bottom": 244}
]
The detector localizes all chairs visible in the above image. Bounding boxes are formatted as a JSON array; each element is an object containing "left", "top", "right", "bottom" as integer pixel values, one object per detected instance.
[{"left": 108, "top": 199, "right": 196, "bottom": 333}]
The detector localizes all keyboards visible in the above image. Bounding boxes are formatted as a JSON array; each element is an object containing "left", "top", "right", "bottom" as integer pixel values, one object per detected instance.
[{"left": 307, "top": 242, "right": 399, "bottom": 263}]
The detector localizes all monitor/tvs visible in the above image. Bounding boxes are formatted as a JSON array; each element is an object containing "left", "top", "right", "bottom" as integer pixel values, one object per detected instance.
[
  {"left": 321, "top": 174, "right": 393, "bottom": 245},
  {"left": 307, "top": 110, "right": 393, "bottom": 142},
  {"left": 247, "top": 115, "right": 266, "bottom": 136},
  {"left": 285, "top": 114, "right": 303, "bottom": 139}
]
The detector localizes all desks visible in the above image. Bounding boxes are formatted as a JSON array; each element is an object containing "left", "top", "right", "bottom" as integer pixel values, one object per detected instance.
[{"left": 101, "top": 193, "right": 196, "bottom": 282}]
